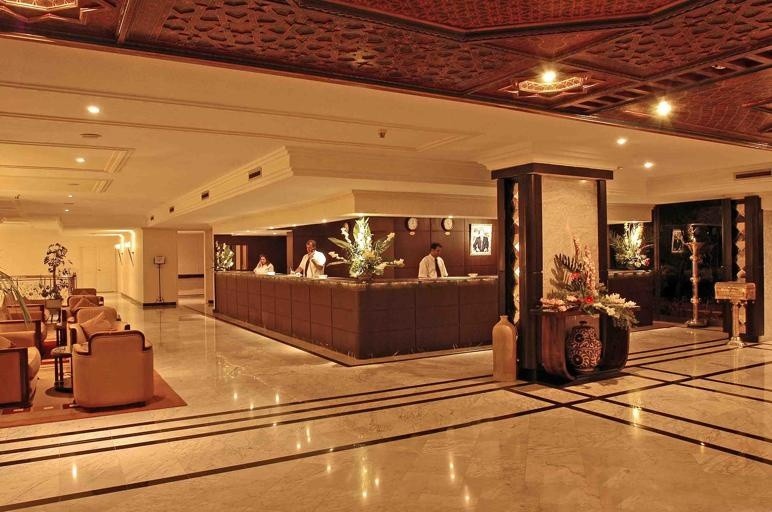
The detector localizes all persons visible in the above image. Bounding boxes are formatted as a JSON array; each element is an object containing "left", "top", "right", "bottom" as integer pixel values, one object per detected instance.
[
  {"left": 473, "top": 228, "right": 489, "bottom": 253},
  {"left": 253, "top": 252, "right": 275, "bottom": 274},
  {"left": 418, "top": 242, "right": 450, "bottom": 278},
  {"left": 295, "top": 240, "right": 327, "bottom": 277}
]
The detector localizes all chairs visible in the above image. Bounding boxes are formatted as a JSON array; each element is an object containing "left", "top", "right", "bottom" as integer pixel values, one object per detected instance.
[{"left": 1, "top": 288, "right": 154, "bottom": 408}]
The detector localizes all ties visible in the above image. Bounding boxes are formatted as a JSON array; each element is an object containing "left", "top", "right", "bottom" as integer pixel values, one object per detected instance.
[
  {"left": 434, "top": 258, "right": 441, "bottom": 278},
  {"left": 303, "top": 257, "right": 311, "bottom": 277}
]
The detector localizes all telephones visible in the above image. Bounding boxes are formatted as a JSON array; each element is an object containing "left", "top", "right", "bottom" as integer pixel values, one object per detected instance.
[{"left": 311, "top": 248, "right": 316, "bottom": 253}]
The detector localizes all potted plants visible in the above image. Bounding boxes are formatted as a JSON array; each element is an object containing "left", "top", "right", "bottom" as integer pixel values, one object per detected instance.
[
  {"left": 608, "top": 221, "right": 653, "bottom": 269},
  {"left": 327, "top": 216, "right": 405, "bottom": 279}
]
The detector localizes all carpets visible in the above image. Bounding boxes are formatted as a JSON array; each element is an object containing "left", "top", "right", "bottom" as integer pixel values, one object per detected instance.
[{"left": 0, "top": 360, "right": 190, "bottom": 429}]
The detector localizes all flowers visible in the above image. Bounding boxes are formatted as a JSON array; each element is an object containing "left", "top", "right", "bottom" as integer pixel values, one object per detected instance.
[{"left": 540, "top": 235, "right": 639, "bottom": 330}]
